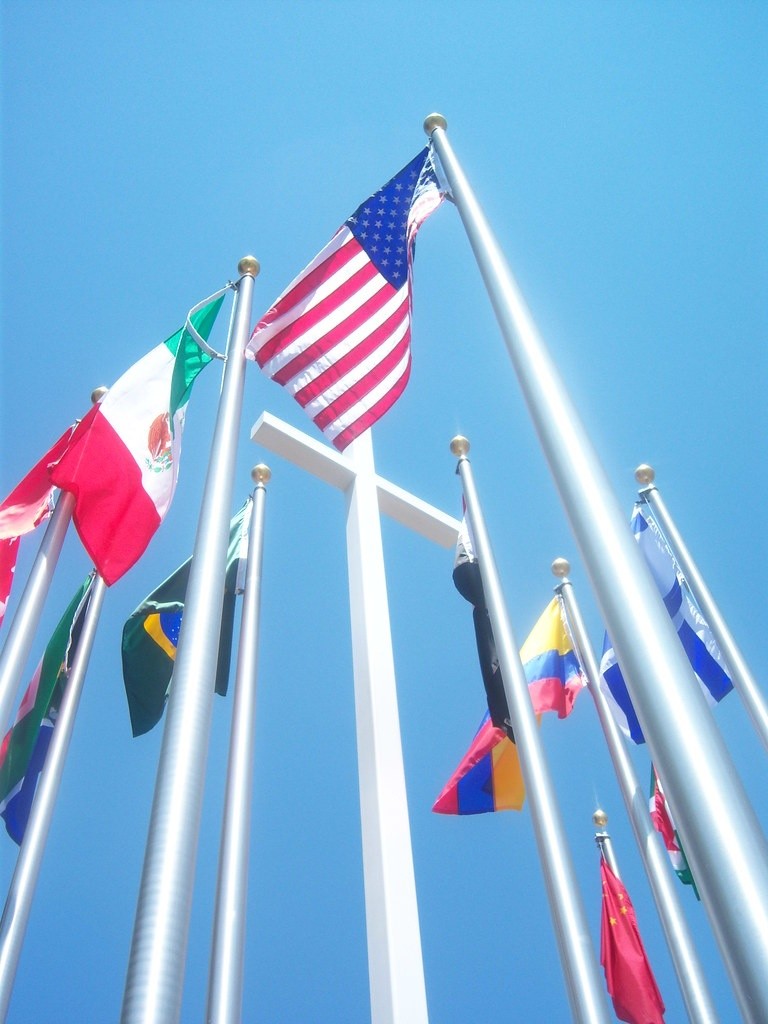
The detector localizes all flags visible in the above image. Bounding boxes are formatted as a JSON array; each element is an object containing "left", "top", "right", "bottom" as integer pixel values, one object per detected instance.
[
  {"left": 599, "top": 502, "right": 737, "bottom": 744},
  {"left": 121, "top": 498, "right": 256, "bottom": 737},
  {"left": 451, "top": 493, "right": 517, "bottom": 746},
  {"left": 600, "top": 854, "right": 665, "bottom": 1023},
  {"left": 0, "top": 576, "right": 114, "bottom": 846},
  {"left": 49, "top": 283, "right": 230, "bottom": 587},
  {"left": 244, "top": 140, "right": 444, "bottom": 453},
  {"left": 648, "top": 760, "right": 704, "bottom": 901},
  {"left": 431, "top": 594, "right": 588, "bottom": 814},
  {"left": 0, "top": 423, "right": 75, "bottom": 628}
]
